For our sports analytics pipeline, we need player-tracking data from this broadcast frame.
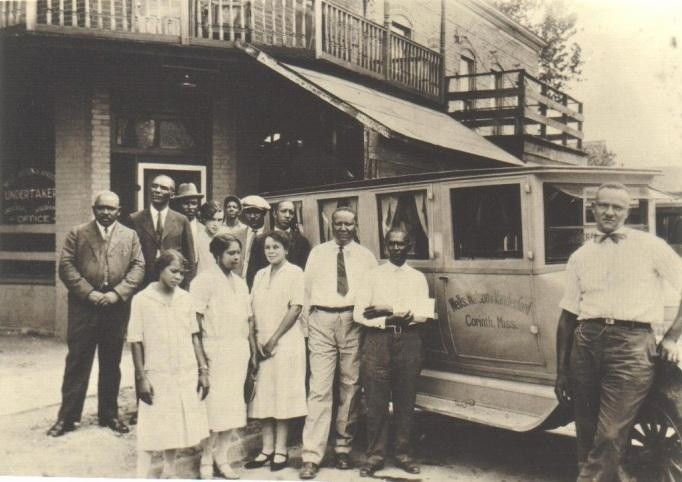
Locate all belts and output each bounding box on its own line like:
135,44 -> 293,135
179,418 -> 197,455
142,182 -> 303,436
581,315 -> 657,328
369,324 -> 423,335
309,303 -> 357,316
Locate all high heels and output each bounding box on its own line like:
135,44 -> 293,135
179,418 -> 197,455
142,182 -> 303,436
244,446 -> 275,471
268,450 -> 290,474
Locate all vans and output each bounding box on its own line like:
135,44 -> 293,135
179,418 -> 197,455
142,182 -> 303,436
244,166 -> 682,480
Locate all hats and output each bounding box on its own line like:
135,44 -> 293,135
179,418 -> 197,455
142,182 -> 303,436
238,194 -> 272,213
222,194 -> 242,215
171,182 -> 205,200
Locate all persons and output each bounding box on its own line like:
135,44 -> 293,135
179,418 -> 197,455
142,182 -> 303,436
555,182 -> 681,481
127,175 -> 378,478
353,226 -> 429,476
47,190 -> 143,437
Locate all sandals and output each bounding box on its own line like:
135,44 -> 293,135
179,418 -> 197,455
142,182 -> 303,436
199,458 -> 240,480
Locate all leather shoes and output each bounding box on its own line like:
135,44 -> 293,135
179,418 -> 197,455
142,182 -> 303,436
392,457 -> 421,475
332,452 -> 354,470
98,412 -> 130,435
298,461 -> 320,481
359,459 -> 385,478
46,420 -> 77,438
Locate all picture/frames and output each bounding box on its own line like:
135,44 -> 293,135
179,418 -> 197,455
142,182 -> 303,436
137,162 -> 207,212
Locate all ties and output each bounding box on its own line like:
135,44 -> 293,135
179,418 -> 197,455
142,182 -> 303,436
245,229 -> 258,264
154,211 -> 163,249
99,226 -> 111,288
336,245 -> 349,299
